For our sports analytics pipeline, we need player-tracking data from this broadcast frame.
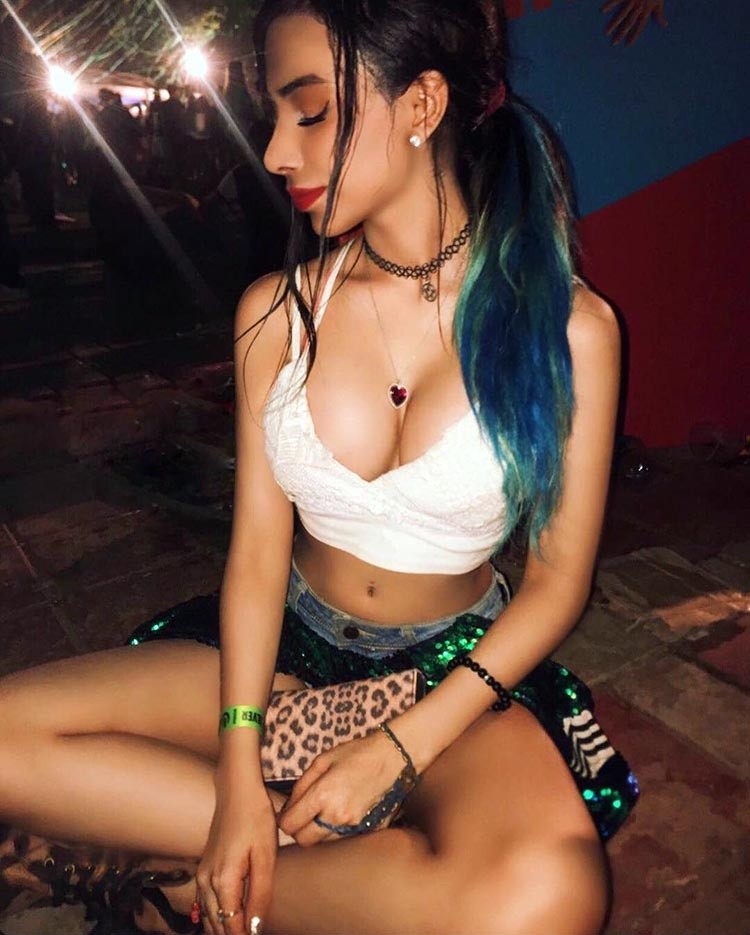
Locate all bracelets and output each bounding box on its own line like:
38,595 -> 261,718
447,655 -> 512,711
217,704 -> 265,735
375,720 -> 421,789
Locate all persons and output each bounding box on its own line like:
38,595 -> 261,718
17,80 -> 221,240
0,1 -> 623,934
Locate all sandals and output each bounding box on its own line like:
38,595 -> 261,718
0,825 -> 203,935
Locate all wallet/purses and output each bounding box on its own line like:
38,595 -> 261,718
259,666 -> 425,790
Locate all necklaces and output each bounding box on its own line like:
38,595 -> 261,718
361,216 -> 475,302
361,237 -> 468,410
